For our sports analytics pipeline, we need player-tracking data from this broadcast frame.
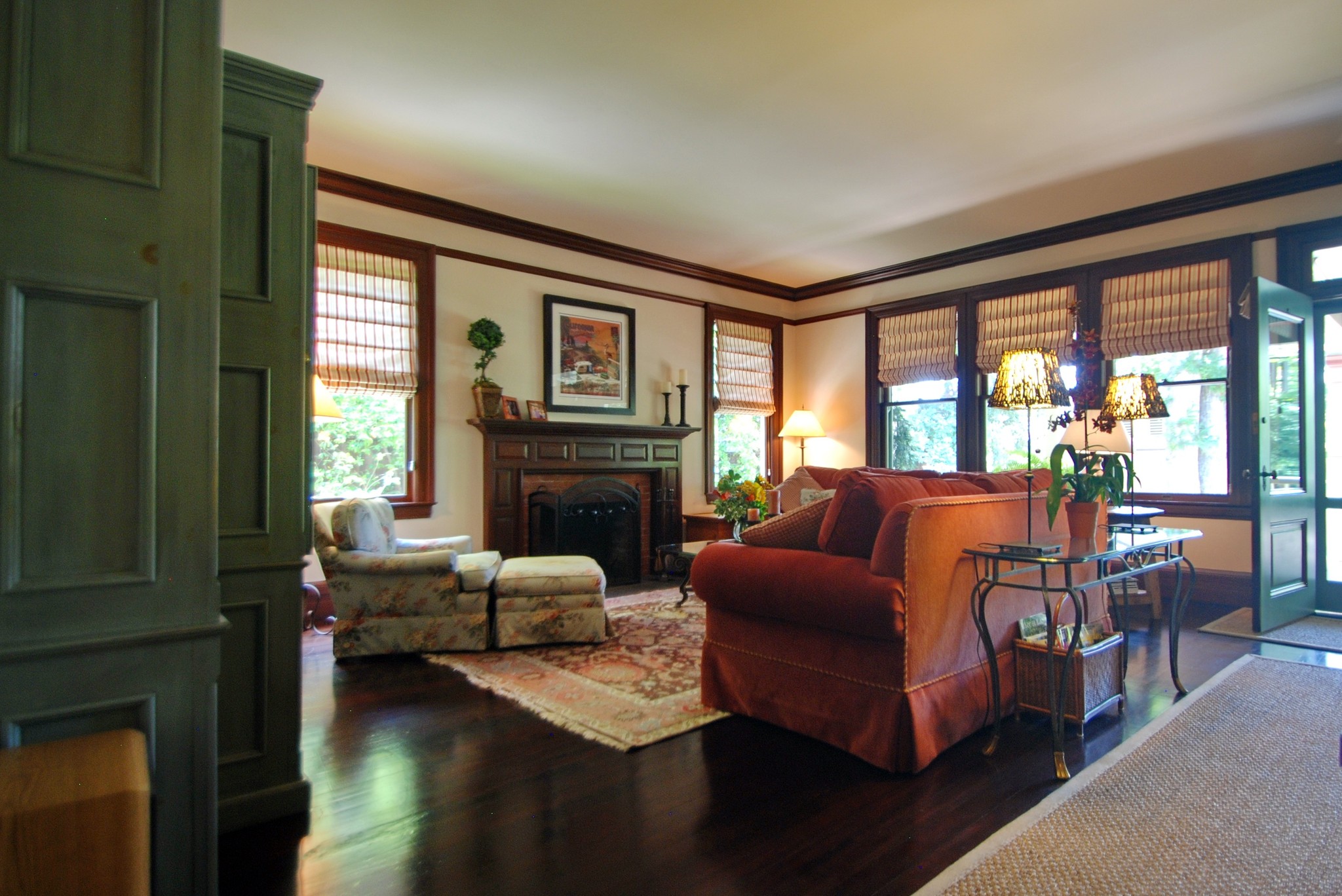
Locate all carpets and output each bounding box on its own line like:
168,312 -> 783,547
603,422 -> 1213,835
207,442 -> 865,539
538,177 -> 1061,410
1196,604 -> 1342,658
416,582 -> 736,754
910,650 -> 1341,896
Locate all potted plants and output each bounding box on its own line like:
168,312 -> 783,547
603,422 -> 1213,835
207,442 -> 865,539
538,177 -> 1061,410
468,314 -> 505,419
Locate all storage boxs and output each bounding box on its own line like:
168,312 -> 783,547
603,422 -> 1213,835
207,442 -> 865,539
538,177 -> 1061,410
1011,629 -> 1127,740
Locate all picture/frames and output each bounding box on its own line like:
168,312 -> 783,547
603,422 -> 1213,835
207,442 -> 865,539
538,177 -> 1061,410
501,396 -> 523,420
527,399 -> 549,422
542,293 -> 636,416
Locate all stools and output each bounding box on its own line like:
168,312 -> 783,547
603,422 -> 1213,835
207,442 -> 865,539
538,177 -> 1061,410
492,555 -> 615,650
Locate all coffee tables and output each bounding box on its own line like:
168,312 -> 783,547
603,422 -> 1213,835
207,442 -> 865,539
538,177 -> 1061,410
658,538 -> 736,608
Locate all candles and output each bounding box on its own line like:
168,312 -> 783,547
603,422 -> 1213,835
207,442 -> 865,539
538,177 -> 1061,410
679,369 -> 688,386
768,490 -> 780,515
747,508 -> 760,521
661,381 -> 672,393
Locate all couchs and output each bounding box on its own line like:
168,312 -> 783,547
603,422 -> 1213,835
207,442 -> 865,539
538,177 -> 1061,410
690,465 -> 1109,777
312,497 -> 501,658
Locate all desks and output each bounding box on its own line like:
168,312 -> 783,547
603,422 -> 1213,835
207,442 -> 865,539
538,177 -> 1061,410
961,525 -> 1205,780
1105,505 -> 1168,632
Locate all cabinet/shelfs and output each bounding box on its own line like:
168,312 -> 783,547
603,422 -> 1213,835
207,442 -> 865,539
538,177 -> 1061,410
683,512 -> 735,543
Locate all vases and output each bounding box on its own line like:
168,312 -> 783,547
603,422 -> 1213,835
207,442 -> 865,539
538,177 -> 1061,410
733,519 -> 751,543
1063,500 -> 1099,538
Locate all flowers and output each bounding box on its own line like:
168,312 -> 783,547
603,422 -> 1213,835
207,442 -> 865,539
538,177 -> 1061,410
1044,283 -> 1141,534
711,469 -> 776,523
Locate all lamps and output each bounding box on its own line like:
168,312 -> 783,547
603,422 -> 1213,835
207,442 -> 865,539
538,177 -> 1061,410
1097,372 -> 1169,533
987,345 -> 1064,554
777,410 -> 827,468
1058,410 -> 1134,476
310,373 -> 344,426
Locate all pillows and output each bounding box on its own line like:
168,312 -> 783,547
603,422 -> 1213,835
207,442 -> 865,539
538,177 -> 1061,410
772,465 -> 824,515
801,488 -> 836,506
739,497 -> 834,552
